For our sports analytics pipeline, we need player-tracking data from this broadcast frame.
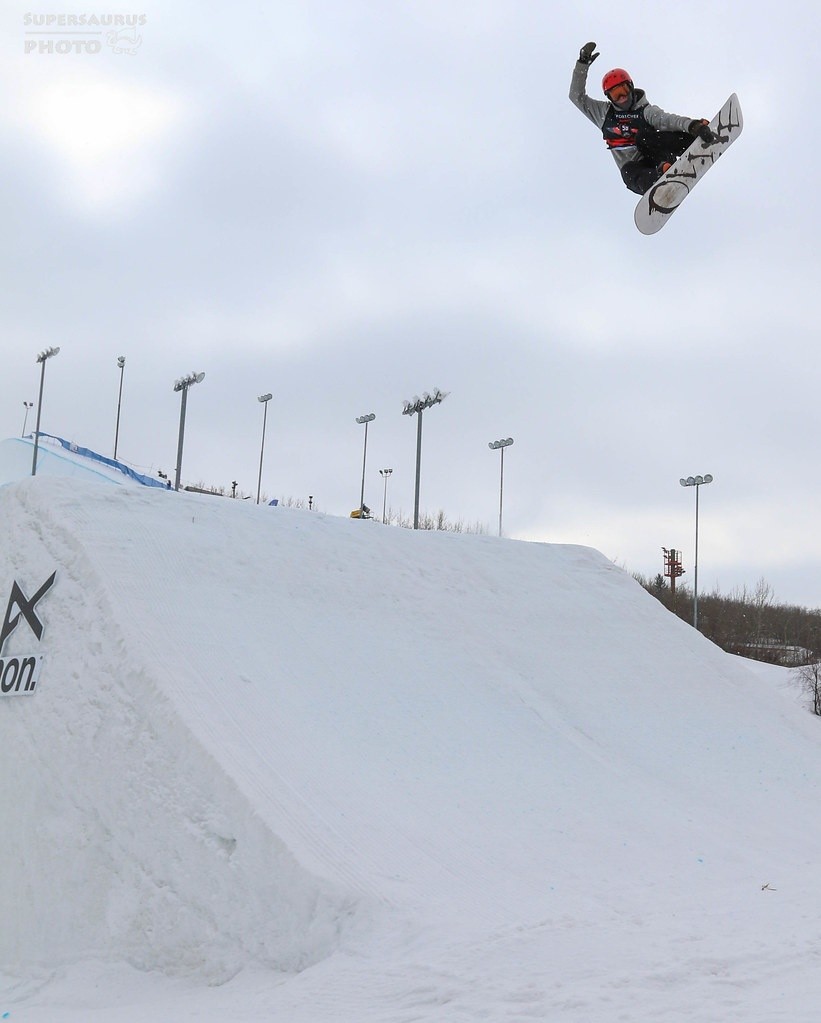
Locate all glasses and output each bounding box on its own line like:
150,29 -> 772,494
608,83 -> 631,102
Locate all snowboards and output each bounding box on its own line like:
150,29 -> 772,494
634,93 -> 744,235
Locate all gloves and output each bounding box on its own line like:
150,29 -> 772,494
577,42 -> 600,65
691,122 -> 714,143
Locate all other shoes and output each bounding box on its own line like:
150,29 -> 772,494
699,118 -> 711,126
656,161 -> 671,178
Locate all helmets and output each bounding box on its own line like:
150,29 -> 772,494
602,67 -> 634,96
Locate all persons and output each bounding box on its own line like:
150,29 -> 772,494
567,41 -> 719,198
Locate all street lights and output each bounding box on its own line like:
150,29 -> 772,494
257,393 -> 272,504
356,414 -> 375,509
22,400 -> 33,437
488,438 -> 514,538
32,346 -> 61,476
679,474 -> 713,626
173,372 -> 206,491
113,356 -> 125,459
402,386 -> 451,530
